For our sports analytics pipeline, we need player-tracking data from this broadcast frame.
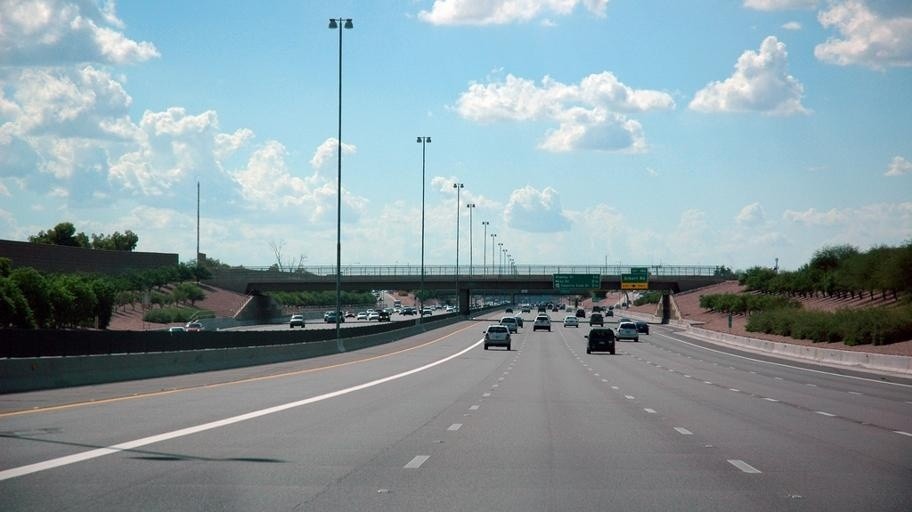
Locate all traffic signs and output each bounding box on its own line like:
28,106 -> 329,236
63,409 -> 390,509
621,274 -> 648,289
631,267 -> 647,274
553,274 -> 602,289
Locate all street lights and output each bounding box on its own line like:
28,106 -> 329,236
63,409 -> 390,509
453,183 -> 464,321
491,233 -> 496,311
481,220 -> 490,314
327,17 -> 355,352
502,247 -> 520,301
497,242 -> 503,309
467,203 -> 475,321
415,136 -> 431,333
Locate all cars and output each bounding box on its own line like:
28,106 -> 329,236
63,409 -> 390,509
289,300 -> 459,328
483,301 -> 649,356
168,327 -> 186,333
185,322 -> 205,332
